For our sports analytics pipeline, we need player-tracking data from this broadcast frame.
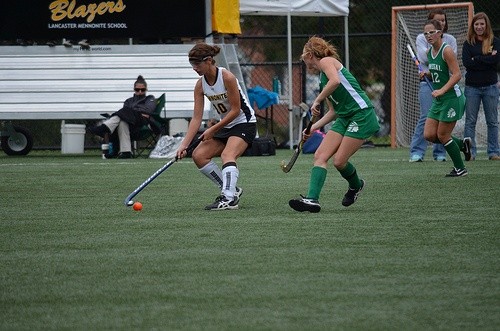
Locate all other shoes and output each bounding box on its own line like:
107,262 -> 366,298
118,152 -> 130,159
85,124 -> 107,138
410,155 -> 423,162
437,157 -> 447,161
489,154 -> 500,160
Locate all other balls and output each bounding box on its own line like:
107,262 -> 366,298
133,202 -> 142,210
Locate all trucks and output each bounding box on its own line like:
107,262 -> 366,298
0,0 -> 249,157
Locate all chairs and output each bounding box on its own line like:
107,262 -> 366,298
132,93 -> 167,158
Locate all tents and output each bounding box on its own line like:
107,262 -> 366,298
213,0 -> 349,148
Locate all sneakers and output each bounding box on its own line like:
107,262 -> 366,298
462,137 -> 472,161
445,167 -> 468,177
205,187 -> 243,211
289,194 -> 321,213
341,179 -> 366,207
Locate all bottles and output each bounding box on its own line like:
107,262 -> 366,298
278,80 -> 281,96
271,76 -> 278,94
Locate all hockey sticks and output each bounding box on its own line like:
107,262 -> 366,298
124,138 -> 202,207
407,43 -> 440,102
280,104 -> 321,174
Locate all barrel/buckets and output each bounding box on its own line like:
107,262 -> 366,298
60,124 -> 86,154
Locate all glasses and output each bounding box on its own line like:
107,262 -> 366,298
134,88 -> 146,92
301,52 -> 311,60
423,30 -> 442,36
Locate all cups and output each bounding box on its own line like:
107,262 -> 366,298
102,144 -> 109,159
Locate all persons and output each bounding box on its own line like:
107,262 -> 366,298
288,38 -> 379,213
304,8 -> 500,176
175,43 -> 258,212
87,75 -> 158,159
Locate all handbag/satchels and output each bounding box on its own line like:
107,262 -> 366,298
248,136 -> 276,157
